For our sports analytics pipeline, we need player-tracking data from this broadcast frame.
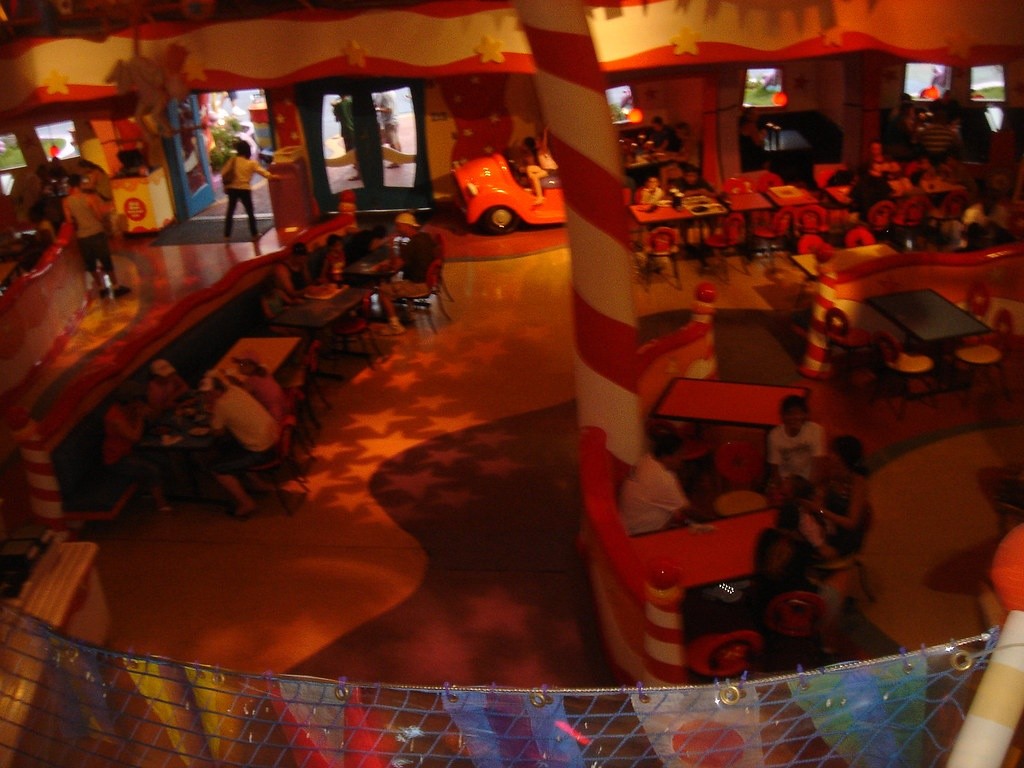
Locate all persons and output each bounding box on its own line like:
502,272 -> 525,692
851,142 -> 900,216
192,349 -> 292,518
765,394 -> 825,501
525,125 -> 552,205
222,141 -> 272,239
0,160 -> 111,261
62,174 -> 130,296
739,107 -> 770,170
749,435 -> 873,625
334,95 -> 363,181
373,91 -> 401,167
624,116 -> 713,250
958,190 -> 1006,252
262,215 -> 437,334
892,89 -> 967,167
617,433 -> 712,534
104,360 -> 187,510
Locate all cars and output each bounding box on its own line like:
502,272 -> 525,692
447,150 -> 633,236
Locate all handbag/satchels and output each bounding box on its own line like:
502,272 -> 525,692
221,159 -> 236,185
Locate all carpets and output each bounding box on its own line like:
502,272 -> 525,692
150,219 -> 273,246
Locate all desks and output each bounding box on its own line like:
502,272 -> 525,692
135,336 -> 303,515
823,179 -> 965,211
765,188 -> 819,251
651,377 -> 812,488
867,288 -> 992,399
1,543 -> 110,768
0,229 -> 37,261
268,286 -> 376,380
721,192 -> 776,260
341,245 -> 430,323
0,262 -> 21,286
628,196 -> 728,269
629,505 -> 808,588
760,128 -> 811,154
626,151 -> 675,168
40,192 -> 69,221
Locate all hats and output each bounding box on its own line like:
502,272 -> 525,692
291,242 -> 307,256
394,212 -> 422,228
150,359 -> 176,378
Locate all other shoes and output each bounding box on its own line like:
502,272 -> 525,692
113,284 -> 130,297
232,500 -> 263,520
99,287 -> 110,298
376,326 -> 406,335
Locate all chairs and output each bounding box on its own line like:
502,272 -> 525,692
687,590 -> 826,681
951,282 -> 1015,406
644,420 -> 768,519
810,500 -> 876,604
824,306 -> 935,421
623,171 -> 827,291
330,290 -> 385,371
237,339 -> 332,518
405,234 -> 455,334
814,162 -> 971,248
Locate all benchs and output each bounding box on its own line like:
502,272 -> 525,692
46,231 -> 373,520
